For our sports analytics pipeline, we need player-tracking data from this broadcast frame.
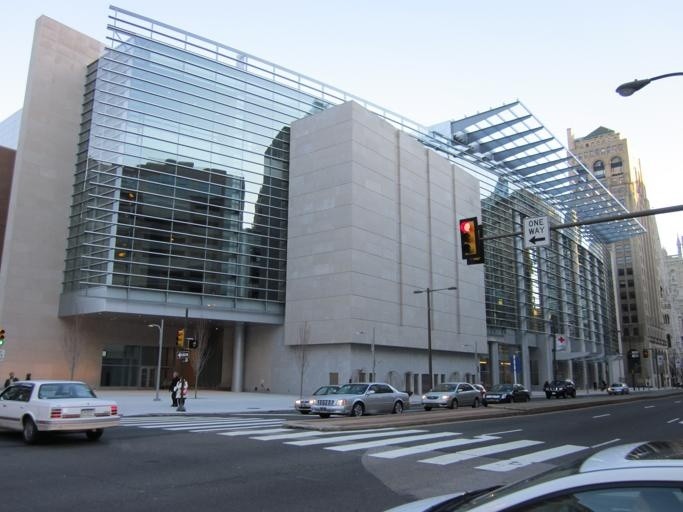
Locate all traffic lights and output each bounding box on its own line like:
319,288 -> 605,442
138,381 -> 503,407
0,329 -> 6,346
189,339 -> 198,348
458,215 -> 479,259
175,328 -> 184,350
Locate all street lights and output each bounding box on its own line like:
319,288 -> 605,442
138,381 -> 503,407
615,70 -> 681,99
413,285 -> 457,389
147,318 -> 164,400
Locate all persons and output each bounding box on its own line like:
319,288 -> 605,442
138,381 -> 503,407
168,371 -> 180,407
544,381 -> 550,400
5,371 -> 19,388
173,377 -> 188,408
26,373 -> 32,380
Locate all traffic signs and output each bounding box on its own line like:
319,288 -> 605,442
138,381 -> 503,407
522,215 -> 550,250
175,350 -> 189,360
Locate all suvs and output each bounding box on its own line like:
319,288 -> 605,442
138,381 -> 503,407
543,378 -> 576,399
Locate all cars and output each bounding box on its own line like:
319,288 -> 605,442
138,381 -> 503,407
379,437 -> 681,512
606,382 -> 628,394
0,379 -> 122,444
310,382 -> 409,418
472,382 -> 486,403
294,384 -> 344,414
482,383 -> 531,404
419,381 -> 483,410
